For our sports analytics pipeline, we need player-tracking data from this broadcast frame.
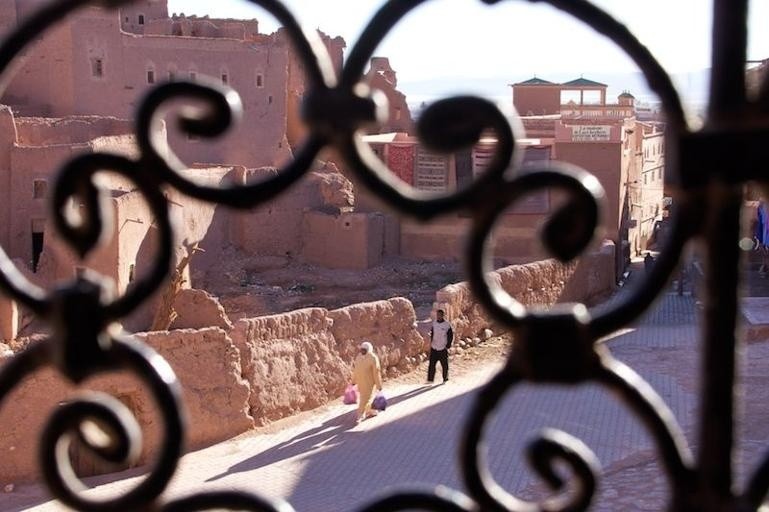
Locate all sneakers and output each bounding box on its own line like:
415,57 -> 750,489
441,379 -> 449,385
354,415 -> 364,426
367,410 -> 379,416
423,381 -> 435,385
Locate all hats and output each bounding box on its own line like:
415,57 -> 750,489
361,340 -> 373,349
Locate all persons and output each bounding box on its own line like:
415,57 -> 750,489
351,341 -> 384,425
643,252 -> 654,273
422,309 -> 453,384
